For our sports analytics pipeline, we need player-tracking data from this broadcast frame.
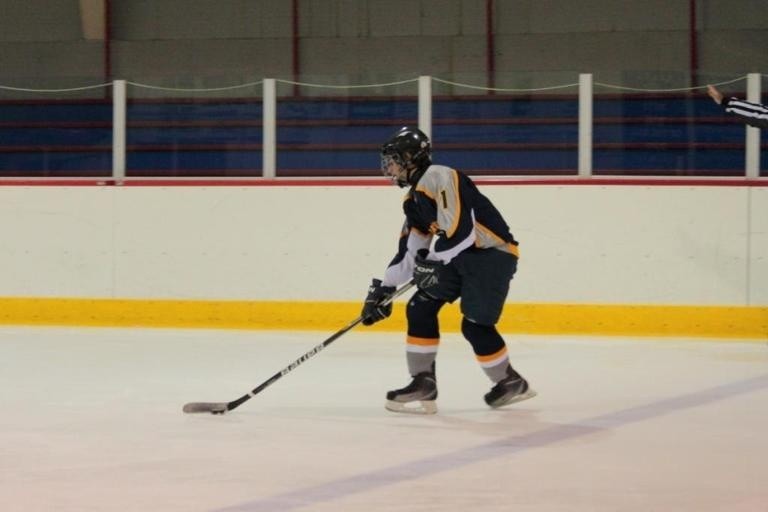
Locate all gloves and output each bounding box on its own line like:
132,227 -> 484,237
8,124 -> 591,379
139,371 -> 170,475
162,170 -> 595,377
361,278 -> 395,325
413,248 -> 443,290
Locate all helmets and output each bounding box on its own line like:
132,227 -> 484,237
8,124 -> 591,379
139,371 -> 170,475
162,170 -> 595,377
379,126 -> 429,182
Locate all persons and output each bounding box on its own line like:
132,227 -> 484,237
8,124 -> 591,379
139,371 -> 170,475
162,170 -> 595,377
706,84 -> 768,130
361,126 -> 528,408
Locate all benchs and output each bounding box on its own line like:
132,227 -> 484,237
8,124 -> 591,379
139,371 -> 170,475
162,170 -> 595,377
1,95 -> 765,175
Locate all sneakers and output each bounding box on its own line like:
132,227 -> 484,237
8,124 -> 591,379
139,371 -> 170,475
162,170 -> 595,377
484,365 -> 527,407
387,361 -> 436,403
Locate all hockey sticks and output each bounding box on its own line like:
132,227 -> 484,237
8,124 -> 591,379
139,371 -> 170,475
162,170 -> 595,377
183,280 -> 413,416
